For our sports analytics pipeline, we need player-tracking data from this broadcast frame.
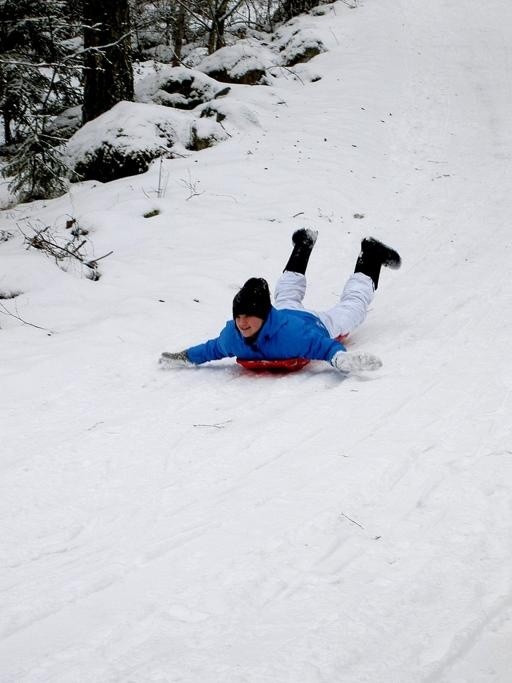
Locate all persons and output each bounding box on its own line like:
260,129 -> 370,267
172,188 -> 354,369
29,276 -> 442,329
151,221 -> 401,376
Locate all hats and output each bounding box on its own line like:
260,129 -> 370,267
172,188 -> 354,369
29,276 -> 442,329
231,276 -> 272,321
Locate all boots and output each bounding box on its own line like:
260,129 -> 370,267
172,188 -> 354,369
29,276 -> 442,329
282,227 -> 320,274
353,236 -> 402,289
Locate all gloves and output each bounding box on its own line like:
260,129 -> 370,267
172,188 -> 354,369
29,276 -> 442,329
161,349 -> 195,369
330,350 -> 384,374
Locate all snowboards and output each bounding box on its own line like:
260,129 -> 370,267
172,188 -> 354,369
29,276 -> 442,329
236,333 -> 350,371
